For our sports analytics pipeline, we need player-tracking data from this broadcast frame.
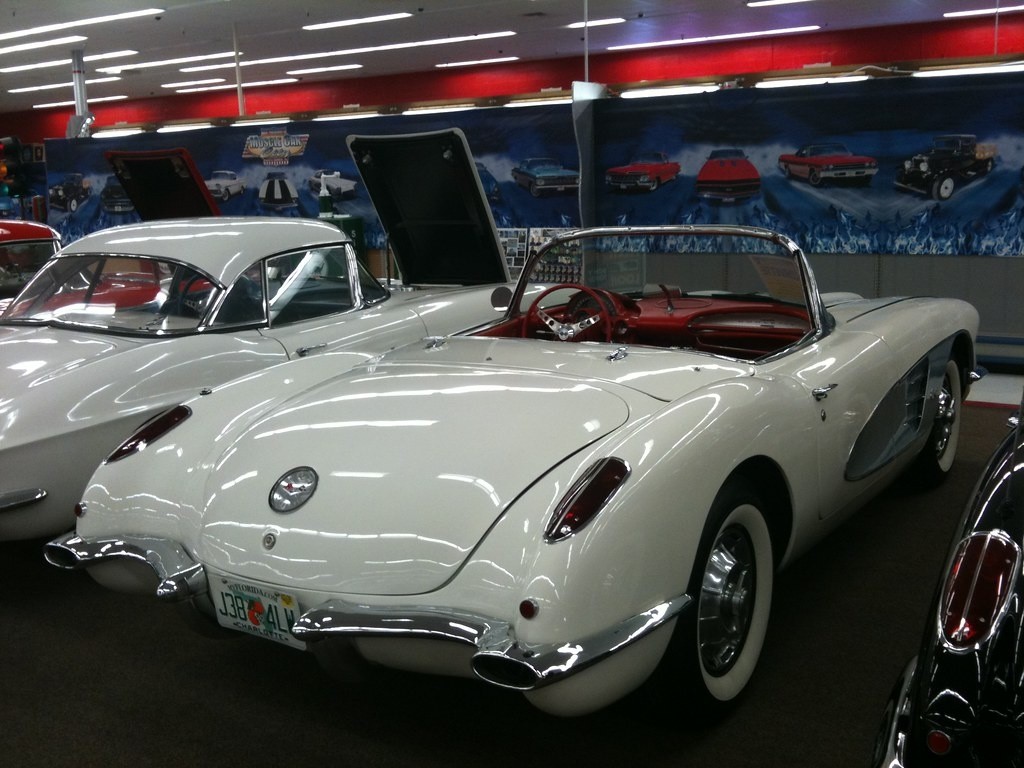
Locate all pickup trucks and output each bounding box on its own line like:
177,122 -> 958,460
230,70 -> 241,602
205,170 -> 247,202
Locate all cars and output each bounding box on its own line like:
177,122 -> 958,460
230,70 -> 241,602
510,157 -> 581,198
891,134 -> 996,203
605,151 -> 681,191
308,168 -> 359,201
0,146 -> 223,321
49,173 -> 94,212
258,172 -> 300,210
777,142 -> 882,188
474,161 -> 502,203
871,392 -> 1024,768
694,148 -> 762,203
100,175 -> 135,213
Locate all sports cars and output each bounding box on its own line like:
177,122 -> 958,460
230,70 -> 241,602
1,126 -> 583,545
42,217 -> 988,727
